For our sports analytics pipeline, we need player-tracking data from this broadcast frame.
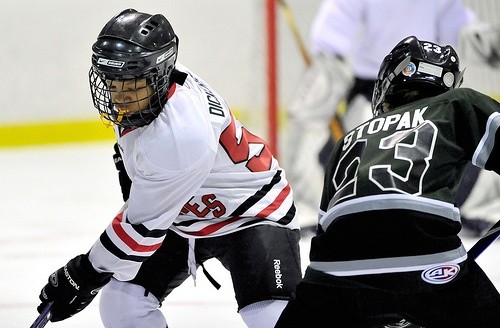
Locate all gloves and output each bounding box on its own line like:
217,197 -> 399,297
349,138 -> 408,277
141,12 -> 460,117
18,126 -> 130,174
37,252 -> 114,323
114,142 -> 131,202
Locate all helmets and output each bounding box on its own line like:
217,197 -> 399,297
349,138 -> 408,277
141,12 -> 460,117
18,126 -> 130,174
371,36 -> 465,118
91,8 -> 178,128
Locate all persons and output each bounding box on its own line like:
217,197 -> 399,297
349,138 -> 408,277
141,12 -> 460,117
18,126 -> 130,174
38,8 -> 302,328
273,37 -> 500,328
306,0 -> 500,173
